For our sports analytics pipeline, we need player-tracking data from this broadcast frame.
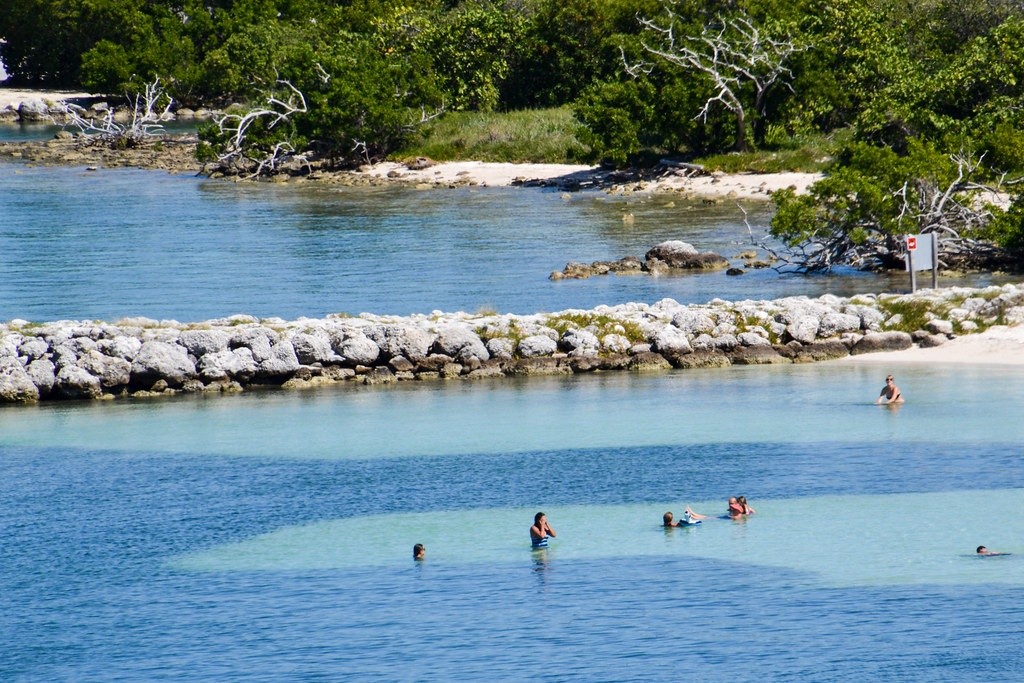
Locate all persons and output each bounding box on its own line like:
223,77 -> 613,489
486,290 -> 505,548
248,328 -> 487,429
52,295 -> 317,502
530,511 -> 556,548
875,375 -> 904,403
413,544 -> 425,559
976,545 -> 991,553
663,496 -> 755,526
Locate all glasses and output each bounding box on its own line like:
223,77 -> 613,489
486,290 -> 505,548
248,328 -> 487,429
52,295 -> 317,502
886,378 -> 893,381
420,548 -> 426,552
